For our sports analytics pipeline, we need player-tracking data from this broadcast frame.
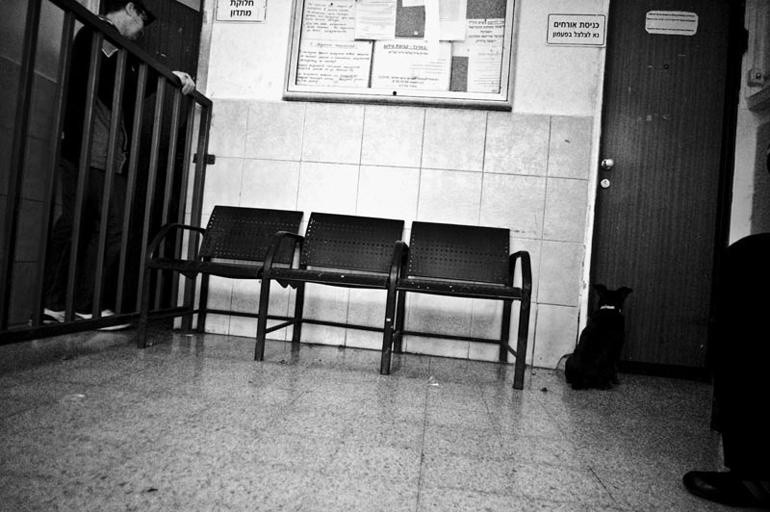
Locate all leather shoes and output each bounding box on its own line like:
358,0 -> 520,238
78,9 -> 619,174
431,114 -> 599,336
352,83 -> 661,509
682,467 -> 770,511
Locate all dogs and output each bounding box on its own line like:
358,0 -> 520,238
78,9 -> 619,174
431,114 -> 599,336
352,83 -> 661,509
564,284 -> 634,391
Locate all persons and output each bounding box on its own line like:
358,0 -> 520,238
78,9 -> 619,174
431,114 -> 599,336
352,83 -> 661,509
25,0 -> 197,331
681,228 -> 769,511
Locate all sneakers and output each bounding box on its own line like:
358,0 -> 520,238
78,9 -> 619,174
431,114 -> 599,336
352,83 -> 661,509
27,307 -> 65,327
74,311 -> 130,332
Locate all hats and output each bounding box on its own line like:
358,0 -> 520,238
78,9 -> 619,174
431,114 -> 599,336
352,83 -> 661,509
102,0 -> 156,26
